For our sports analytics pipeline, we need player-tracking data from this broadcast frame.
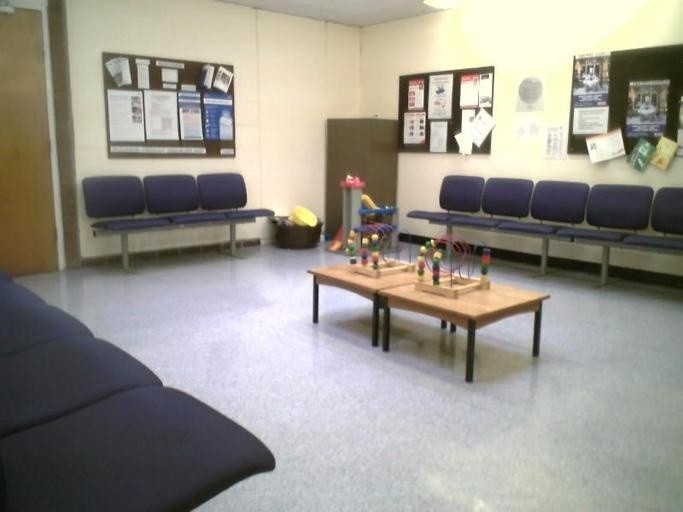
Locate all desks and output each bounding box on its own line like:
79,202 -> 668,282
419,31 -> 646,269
376,271 -> 550,382
306,257 -> 456,347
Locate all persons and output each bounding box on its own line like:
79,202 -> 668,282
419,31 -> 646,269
581,66 -> 605,94
635,95 -> 660,123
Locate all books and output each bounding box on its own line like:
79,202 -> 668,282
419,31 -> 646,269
651,136 -> 678,170
626,137 -> 657,171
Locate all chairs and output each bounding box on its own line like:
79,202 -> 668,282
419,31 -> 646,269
405,174 -> 682,289
82,172 -> 275,275
0,268 -> 275,511
